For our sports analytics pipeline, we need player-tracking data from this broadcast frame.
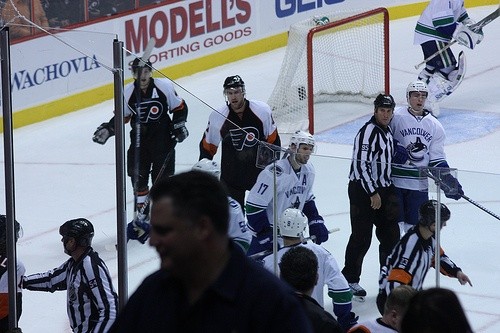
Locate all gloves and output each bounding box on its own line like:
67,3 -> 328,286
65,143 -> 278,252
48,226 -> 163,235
338,312 -> 359,329
441,175 -> 464,200
126,220 -> 149,245
93,122 -> 116,144
308,216 -> 329,245
259,231 -> 279,251
172,121 -> 189,142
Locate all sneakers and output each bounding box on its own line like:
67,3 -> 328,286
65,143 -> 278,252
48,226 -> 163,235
348,282 -> 366,302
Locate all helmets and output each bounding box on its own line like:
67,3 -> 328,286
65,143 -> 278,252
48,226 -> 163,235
406,82 -> 429,94
291,131 -> 315,146
374,93 -> 396,106
0,216 -> 20,244
131,58 -> 152,71
192,158 -> 221,178
420,200 -> 451,220
59,218 -> 94,240
280,208 -> 308,237
224,76 -> 245,90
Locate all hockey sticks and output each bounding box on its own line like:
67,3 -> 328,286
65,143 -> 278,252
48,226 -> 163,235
409,161 -> 500,220
414,6 -> 500,72
249,227 -> 340,260
134,135 -> 180,232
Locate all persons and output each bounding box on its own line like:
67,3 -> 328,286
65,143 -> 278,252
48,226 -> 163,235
0,215 -> 24,333
108,170 -> 311,332
377,199 -> 473,317
196,73 -> 280,202
189,159 -> 252,256
380,81 -> 462,234
342,94 -> 400,304
271,248 -> 338,333
21,217 -> 118,333
91,56 -> 189,225
415,1 -> 484,101
401,288 -> 473,333
346,285 -> 416,333
260,209 -> 353,320
244,128 -> 330,259
